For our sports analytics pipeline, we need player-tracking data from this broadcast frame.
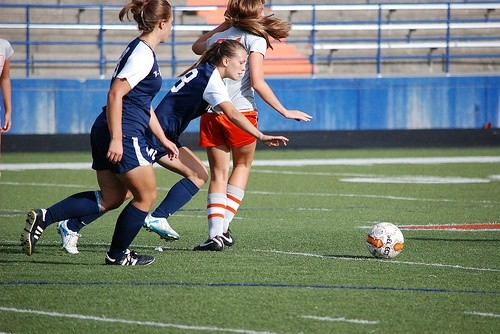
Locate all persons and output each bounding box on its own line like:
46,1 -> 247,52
57,39 -> 290,254
0,36 -> 15,135
192,0 -> 313,251
19,0 -> 183,268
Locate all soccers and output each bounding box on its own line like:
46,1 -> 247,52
367,222 -> 404,259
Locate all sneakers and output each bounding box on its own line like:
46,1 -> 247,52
106,249 -> 156,266
20,208 -> 47,256
191,235 -> 225,251
143,213 -> 180,243
56,219 -> 83,255
221,228 -> 235,246
